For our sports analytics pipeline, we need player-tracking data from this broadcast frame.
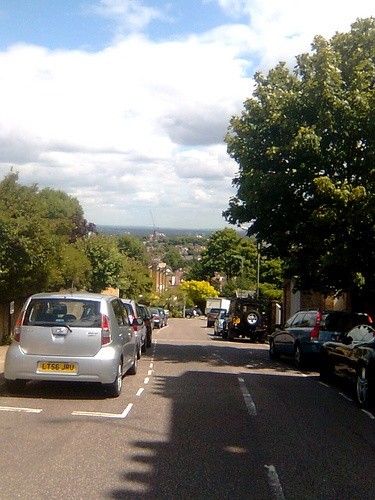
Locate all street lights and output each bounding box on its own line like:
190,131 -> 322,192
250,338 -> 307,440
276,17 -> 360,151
241,226 -> 261,298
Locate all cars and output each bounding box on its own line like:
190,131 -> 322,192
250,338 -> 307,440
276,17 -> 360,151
4,293 -> 137,396
205,297 -> 231,335
185,308 -> 201,318
119,298 -> 169,359
319,323 -> 375,408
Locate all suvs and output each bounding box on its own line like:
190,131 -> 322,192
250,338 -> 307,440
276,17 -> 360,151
270,308 -> 375,371
222,298 -> 272,343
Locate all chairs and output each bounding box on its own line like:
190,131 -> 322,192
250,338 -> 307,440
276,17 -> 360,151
35,312 -> 56,322
62,315 -> 76,322
